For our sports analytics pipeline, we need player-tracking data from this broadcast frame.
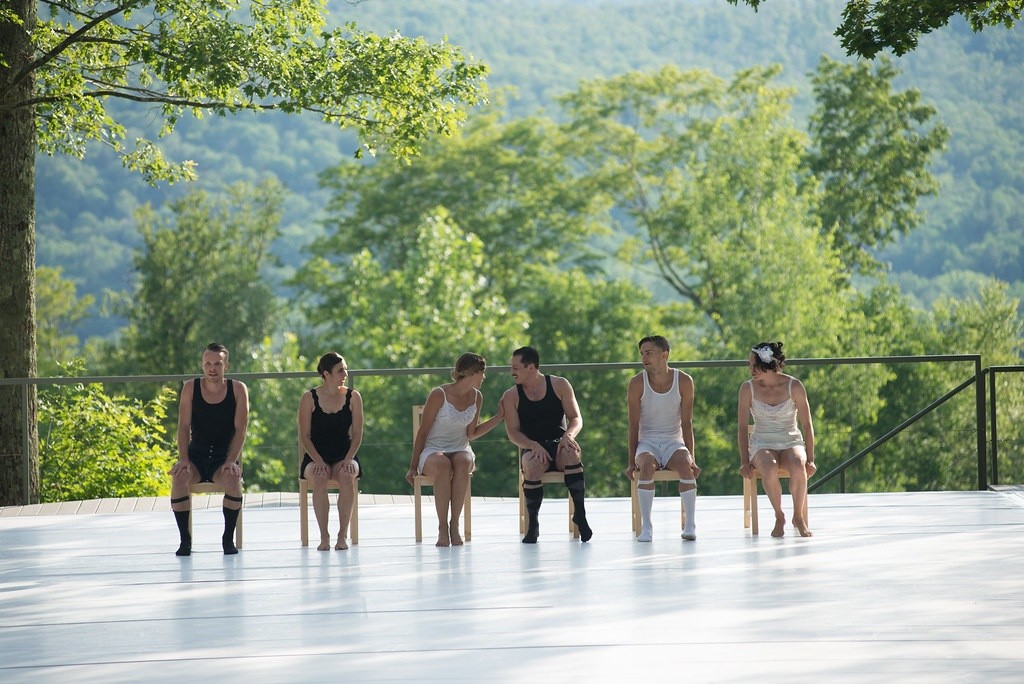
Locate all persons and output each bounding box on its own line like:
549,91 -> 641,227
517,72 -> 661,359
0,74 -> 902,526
405,352 -> 506,548
298,352 -> 364,551
166,342 -> 250,556
737,340 -> 817,538
502,345 -> 593,543
623,335 -> 701,542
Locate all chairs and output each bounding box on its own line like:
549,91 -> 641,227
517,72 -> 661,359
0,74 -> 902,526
518,446 -> 580,539
627,468 -> 696,538
742,423 -> 810,535
297,409 -> 361,546
183,445 -> 245,549
411,405 -> 474,543
